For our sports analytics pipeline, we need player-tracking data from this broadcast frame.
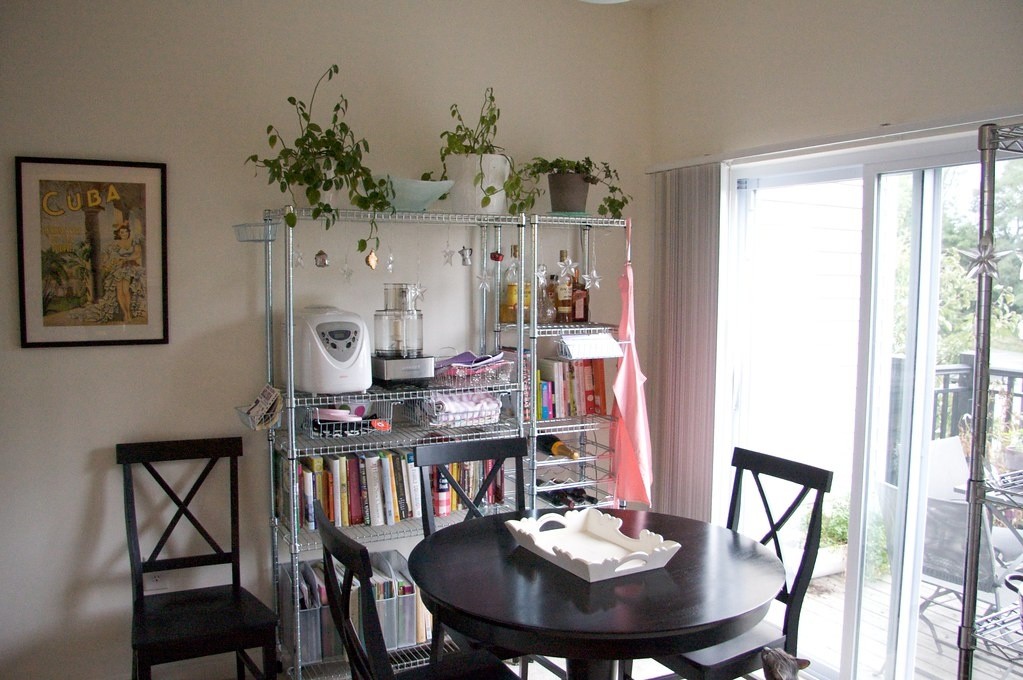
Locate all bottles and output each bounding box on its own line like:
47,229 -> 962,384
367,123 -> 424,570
500,244 -> 589,324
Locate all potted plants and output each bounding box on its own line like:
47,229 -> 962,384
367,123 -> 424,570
421,87 -> 546,215
244,65 -> 397,252
522,156 -> 633,221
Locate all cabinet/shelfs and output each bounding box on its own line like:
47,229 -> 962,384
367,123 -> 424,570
231,205 -> 629,680
957,122 -> 1022,679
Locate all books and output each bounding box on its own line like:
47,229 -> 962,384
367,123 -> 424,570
274,440 -> 513,536
280,560 -> 433,664
499,345 -> 612,422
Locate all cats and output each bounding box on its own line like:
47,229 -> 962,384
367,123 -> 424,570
761,645 -> 810,680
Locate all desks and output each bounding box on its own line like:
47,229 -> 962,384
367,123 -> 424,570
408,508 -> 787,680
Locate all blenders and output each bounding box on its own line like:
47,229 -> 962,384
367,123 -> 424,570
373,282 -> 435,385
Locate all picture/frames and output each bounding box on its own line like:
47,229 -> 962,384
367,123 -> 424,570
13,155 -> 168,348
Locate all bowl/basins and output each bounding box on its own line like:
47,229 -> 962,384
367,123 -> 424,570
361,172 -> 456,212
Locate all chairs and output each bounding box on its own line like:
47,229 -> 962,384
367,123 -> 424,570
878,479 -> 1023,619
413,437 -> 569,680
116,438 -> 279,679
319,522 -> 523,680
617,447 -> 832,680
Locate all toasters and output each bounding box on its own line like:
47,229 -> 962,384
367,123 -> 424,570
279,304 -> 373,396
